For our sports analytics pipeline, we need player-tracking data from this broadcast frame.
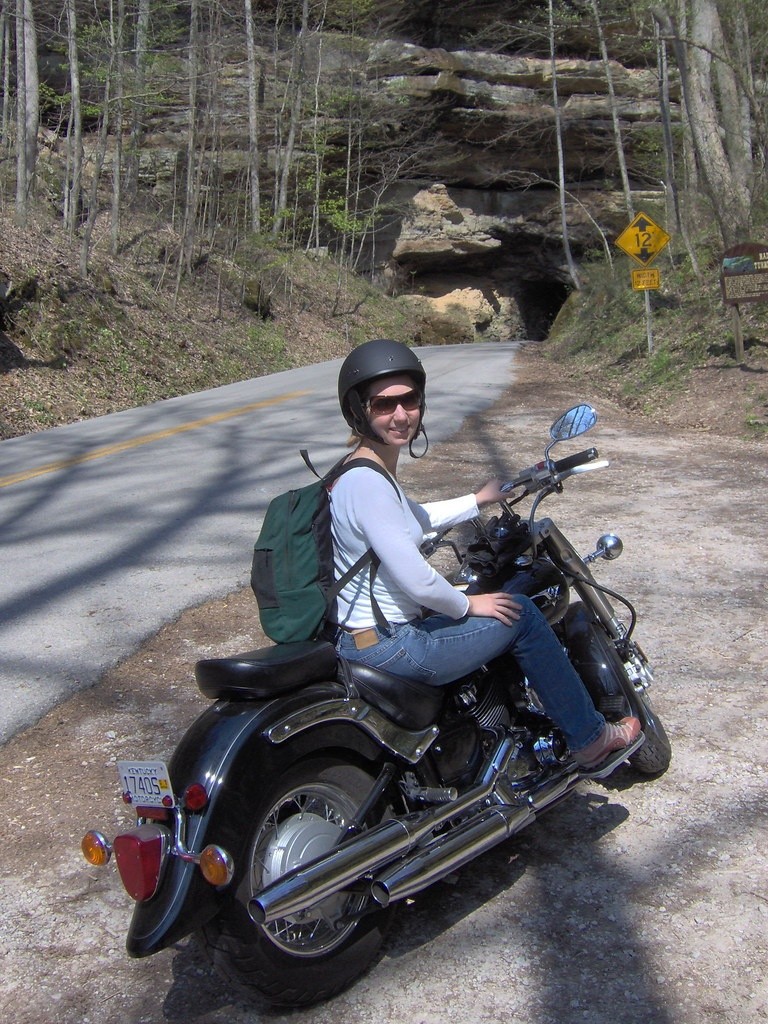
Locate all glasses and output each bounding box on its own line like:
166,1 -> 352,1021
364,385 -> 422,415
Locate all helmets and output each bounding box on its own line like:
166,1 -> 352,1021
338,339 -> 426,429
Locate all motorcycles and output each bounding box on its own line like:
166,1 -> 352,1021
81,403 -> 672,1006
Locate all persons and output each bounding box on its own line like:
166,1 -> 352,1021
322,340 -> 640,769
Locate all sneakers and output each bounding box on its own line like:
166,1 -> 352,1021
572,717 -> 641,770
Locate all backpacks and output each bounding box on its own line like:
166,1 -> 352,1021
250,450 -> 402,645
